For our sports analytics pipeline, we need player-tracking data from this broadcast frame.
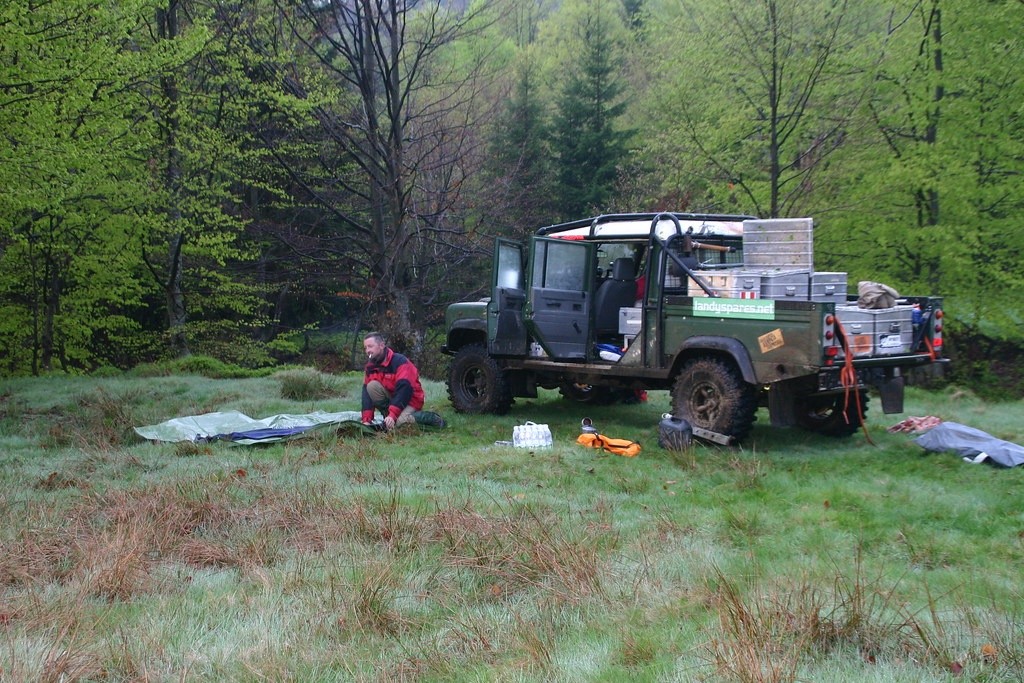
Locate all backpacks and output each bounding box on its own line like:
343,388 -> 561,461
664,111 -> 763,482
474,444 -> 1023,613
575,434 -> 642,458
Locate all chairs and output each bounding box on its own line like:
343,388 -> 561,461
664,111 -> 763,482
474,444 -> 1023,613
594,257 -> 638,337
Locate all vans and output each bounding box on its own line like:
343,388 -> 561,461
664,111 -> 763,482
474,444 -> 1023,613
535,210 -> 762,297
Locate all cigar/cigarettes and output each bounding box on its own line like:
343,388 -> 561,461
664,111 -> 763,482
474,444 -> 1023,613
369,353 -> 372,359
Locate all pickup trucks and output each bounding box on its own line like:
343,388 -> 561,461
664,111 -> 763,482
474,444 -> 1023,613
437,210 -> 954,447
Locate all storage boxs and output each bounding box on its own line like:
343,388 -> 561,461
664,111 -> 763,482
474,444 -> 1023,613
688,217 -> 913,359
619,307 -> 642,335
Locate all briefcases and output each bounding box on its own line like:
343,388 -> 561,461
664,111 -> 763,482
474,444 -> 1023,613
512,421 -> 553,452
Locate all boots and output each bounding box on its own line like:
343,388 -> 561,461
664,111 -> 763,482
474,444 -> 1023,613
375,399 -> 390,419
411,411 -> 446,431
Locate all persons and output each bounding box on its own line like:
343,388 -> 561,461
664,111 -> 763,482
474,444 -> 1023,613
357,332 -> 445,432
